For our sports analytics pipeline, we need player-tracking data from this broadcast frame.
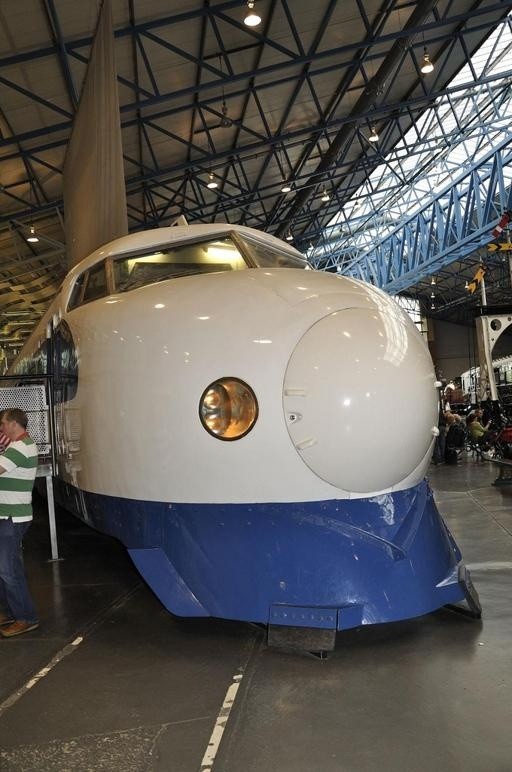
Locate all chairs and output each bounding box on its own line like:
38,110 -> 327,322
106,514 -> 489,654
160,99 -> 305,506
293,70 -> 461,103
436,395 -> 512,463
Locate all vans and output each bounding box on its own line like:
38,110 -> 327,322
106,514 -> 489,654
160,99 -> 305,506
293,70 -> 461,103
446,401 -> 478,417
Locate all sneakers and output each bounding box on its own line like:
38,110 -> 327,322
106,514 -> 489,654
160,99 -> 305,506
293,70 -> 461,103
0,612 -> 16,625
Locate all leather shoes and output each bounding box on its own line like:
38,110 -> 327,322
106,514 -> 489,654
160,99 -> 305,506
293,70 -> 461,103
0,617 -> 41,638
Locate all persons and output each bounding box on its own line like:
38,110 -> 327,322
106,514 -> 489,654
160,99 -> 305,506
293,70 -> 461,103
0,408 -> 43,636
433,403 -> 496,464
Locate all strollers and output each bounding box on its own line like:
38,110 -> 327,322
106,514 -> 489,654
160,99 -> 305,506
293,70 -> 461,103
482,402 -> 512,459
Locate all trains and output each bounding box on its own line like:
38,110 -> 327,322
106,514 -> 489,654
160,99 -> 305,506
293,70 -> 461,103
0,214 -> 480,660
